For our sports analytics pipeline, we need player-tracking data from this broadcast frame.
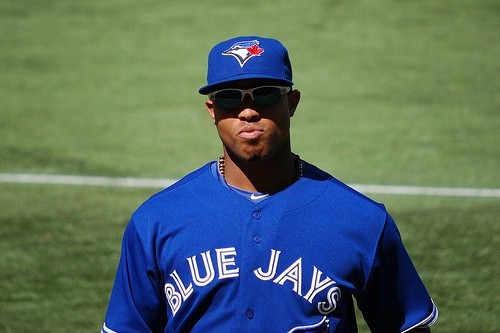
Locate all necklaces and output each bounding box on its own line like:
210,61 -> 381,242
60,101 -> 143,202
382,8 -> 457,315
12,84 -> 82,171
217,152 -> 307,191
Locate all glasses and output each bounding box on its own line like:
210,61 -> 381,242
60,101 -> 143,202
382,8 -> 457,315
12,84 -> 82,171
206,84 -> 289,107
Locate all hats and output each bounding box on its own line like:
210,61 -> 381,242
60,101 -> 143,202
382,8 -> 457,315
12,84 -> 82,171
197,36 -> 297,94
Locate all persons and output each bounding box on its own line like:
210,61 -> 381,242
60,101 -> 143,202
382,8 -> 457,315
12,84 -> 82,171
100,36 -> 441,333
